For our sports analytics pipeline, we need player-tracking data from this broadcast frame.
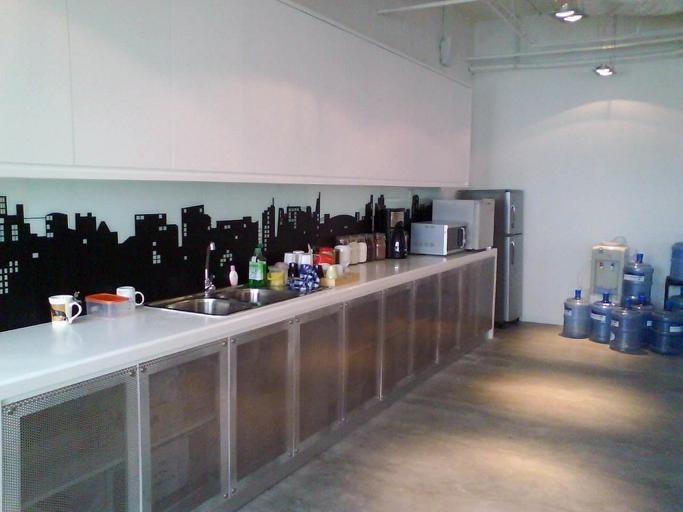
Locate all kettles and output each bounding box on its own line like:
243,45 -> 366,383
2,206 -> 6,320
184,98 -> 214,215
388,220 -> 408,259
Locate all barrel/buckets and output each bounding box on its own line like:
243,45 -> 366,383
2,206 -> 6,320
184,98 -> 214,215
590,292 -> 619,343
622,253 -> 655,306
563,289 -> 592,338
669,242 -> 683,283
669,287 -> 683,310
629,295 -> 656,347
649,299 -> 683,355
609,296 -> 643,353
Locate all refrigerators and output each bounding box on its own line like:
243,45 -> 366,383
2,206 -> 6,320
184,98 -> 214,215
456,186 -> 522,328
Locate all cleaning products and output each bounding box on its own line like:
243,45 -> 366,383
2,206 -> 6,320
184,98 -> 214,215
247,245 -> 268,288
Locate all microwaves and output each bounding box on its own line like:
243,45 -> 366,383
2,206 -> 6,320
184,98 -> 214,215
409,219 -> 468,258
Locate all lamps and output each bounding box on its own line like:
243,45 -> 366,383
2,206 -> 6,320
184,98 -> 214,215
592,62 -> 614,77
551,1 -> 585,23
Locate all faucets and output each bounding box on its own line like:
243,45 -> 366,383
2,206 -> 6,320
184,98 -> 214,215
203,241 -> 218,296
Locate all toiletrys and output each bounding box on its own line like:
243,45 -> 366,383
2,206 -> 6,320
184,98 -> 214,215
229,265 -> 238,286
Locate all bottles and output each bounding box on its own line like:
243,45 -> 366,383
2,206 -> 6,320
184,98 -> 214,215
228,265 -> 238,287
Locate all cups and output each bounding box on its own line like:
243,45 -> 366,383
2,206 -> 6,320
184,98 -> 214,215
48,294 -> 82,329
115,285 -> 144,313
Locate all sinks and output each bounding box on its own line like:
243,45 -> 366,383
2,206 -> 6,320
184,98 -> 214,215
217,285 -> 301,307
145,296 -> 255,317
